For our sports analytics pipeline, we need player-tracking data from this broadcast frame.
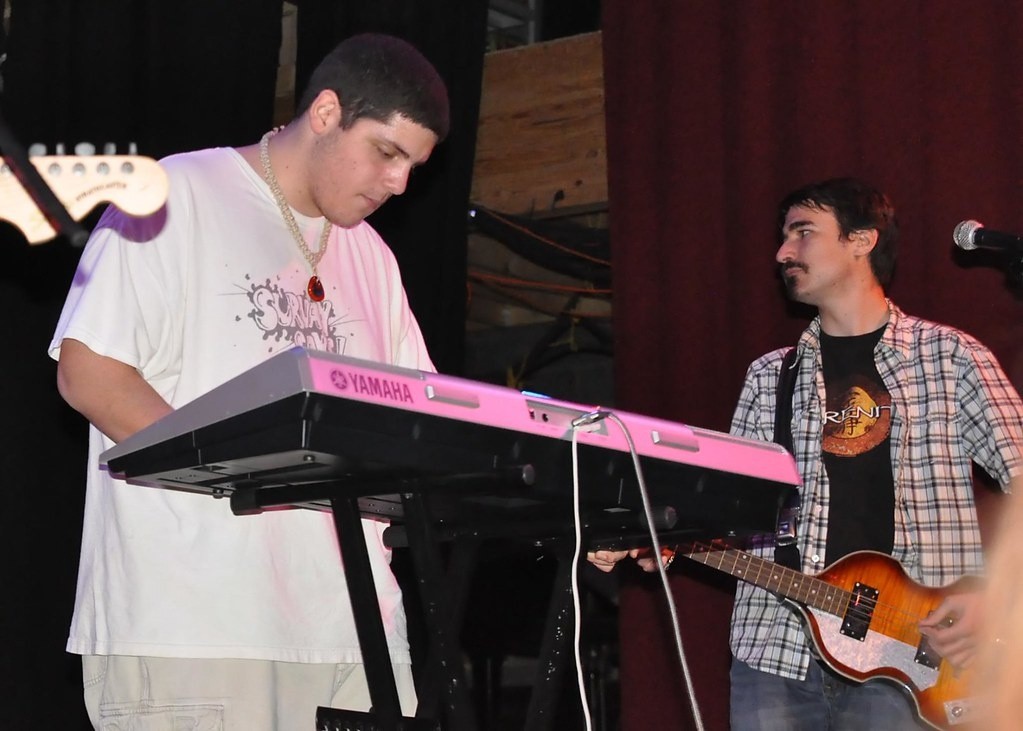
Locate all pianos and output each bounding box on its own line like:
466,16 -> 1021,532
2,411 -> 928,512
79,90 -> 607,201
98,345 -> 800,559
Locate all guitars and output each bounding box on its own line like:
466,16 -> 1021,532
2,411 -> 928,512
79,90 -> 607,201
640,534 -> 1023,731
0,144 -> 166,246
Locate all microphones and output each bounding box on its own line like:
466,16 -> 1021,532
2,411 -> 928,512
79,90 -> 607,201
952,220 -> 1023,255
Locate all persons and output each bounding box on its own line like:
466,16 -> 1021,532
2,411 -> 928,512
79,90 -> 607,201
585,175 -> 1023,731
47,32 -> 451,731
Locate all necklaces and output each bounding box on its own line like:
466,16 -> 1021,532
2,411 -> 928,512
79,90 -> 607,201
260,124 -> 334,303
